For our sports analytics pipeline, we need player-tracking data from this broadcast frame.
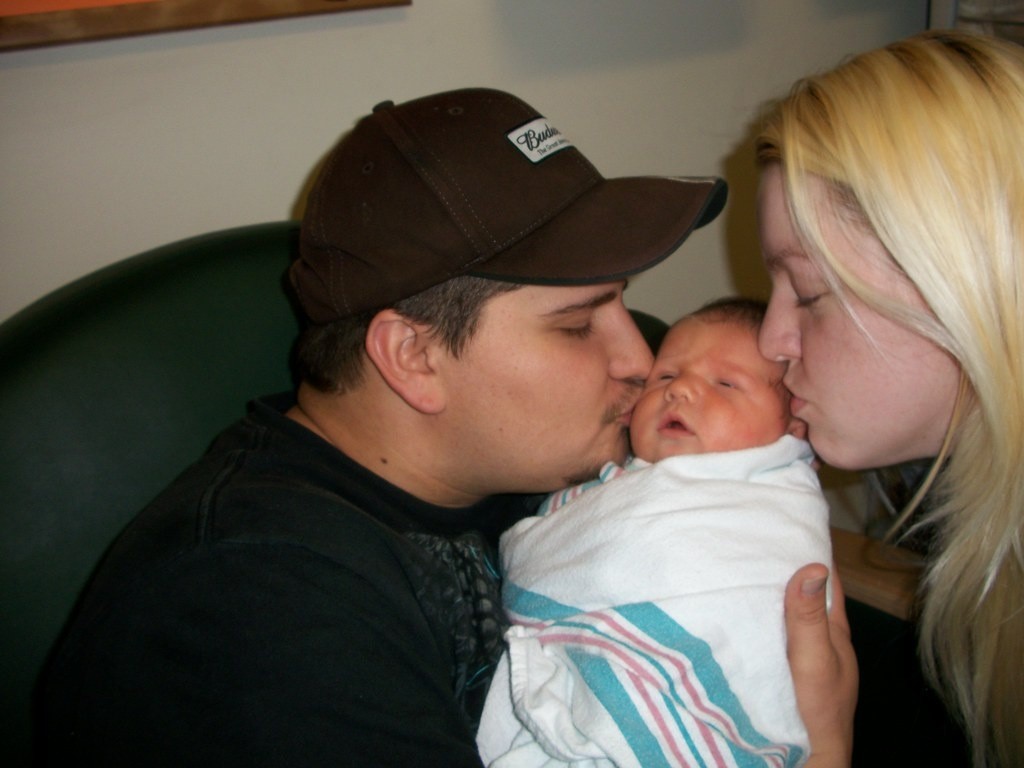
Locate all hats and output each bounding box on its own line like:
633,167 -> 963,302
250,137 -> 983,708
289,88 -> 728,326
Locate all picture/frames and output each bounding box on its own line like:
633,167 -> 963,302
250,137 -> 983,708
0,0 -> 413,54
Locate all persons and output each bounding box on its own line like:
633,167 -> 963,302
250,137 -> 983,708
741,30 -> 1024,768
32,89 -> 859,767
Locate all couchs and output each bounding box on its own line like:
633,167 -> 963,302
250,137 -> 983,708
0,219 -> 671,768
